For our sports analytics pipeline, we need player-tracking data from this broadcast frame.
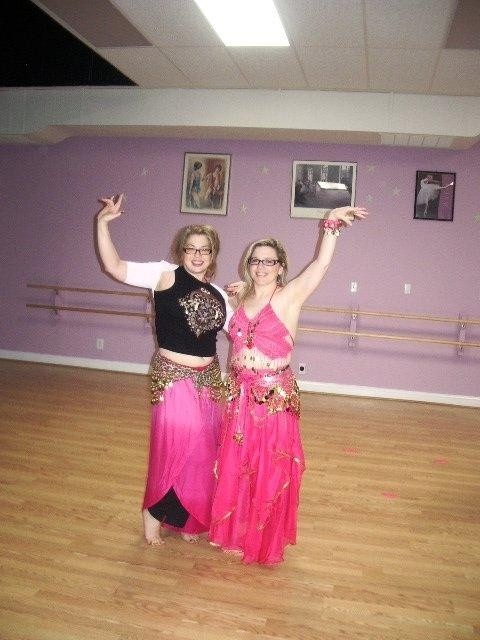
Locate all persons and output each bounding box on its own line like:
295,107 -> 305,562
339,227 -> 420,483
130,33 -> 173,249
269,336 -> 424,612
206,205 -> 368,569
95,193 -> 234,549
206,164 -> 223,210
188,161 -> 204,210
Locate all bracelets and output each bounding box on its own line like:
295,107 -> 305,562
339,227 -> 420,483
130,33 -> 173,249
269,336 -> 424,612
322,219 -> 342,237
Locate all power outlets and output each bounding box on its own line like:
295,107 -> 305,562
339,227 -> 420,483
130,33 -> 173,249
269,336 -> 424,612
351,282 -> 357,293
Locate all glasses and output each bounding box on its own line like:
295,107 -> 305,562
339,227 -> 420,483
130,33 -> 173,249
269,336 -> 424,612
183,247 -> 212,255
248,258 -> 281,266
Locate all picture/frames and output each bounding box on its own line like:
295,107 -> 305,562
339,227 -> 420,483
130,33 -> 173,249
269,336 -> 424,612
414,170 -> 456,222
180,152 -> 232,217
290,160 -> 357,221
